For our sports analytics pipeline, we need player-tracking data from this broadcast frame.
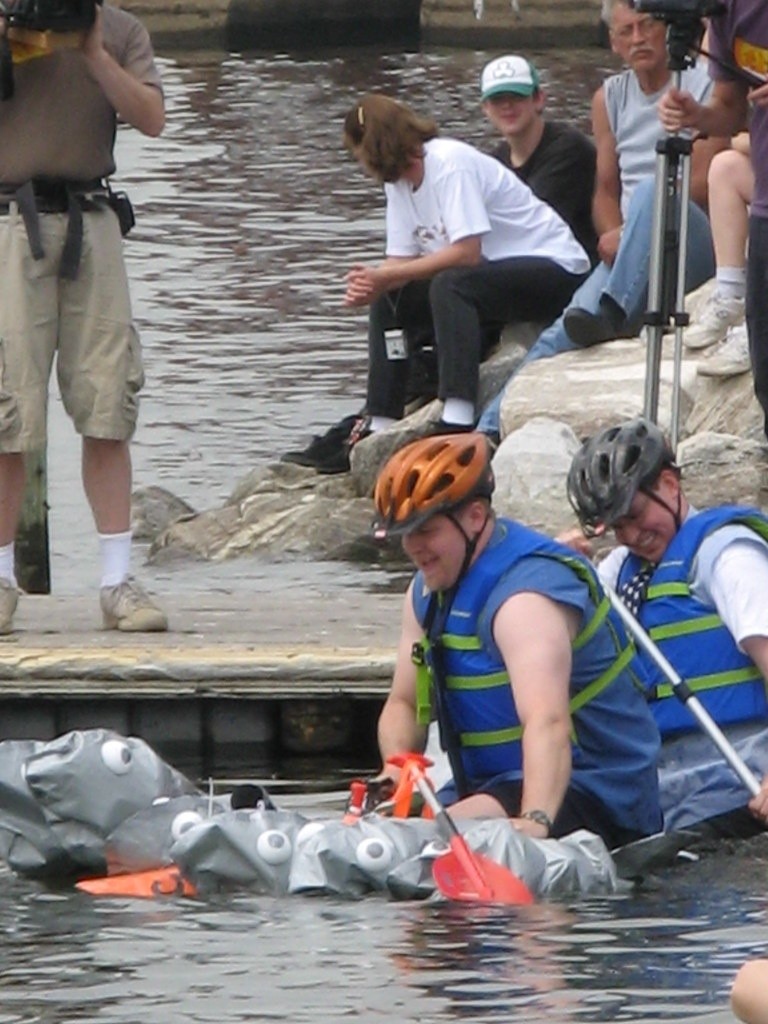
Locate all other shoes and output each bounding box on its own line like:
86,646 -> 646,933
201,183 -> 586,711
316,429 -> 375,473
280,432 -> 345,465
682,291 -> 749,350
697,323 -> 753,376
428,419 -> 475,435
564,308 -> 617,348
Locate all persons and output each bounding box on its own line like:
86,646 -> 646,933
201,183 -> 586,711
342,431 -> 663,849
564,420 -> 768,830
278,52 -> 597,472
0,1 -> 169,634
472,0 -> 715,442
316,91 -> 591,473
660,1 -> 767,440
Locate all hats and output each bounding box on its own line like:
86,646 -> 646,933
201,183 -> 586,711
479,53 -> 540,102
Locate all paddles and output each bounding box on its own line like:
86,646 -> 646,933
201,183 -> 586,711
600,583 -> 764,800
407,760 -> 534,905
74,759 -> 411,897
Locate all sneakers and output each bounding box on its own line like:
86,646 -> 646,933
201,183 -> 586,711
0,578 -> 19,635
100,574 -> 167,632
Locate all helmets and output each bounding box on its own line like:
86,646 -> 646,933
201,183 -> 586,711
566,418 -> 674,538
371,433 -> 488,539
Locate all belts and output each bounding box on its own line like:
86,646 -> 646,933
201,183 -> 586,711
0,199 -> 88,214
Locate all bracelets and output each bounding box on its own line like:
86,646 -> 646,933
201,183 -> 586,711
522,804 -> 554,836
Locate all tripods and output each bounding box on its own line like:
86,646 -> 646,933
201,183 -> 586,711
640,11 -> 768,463
